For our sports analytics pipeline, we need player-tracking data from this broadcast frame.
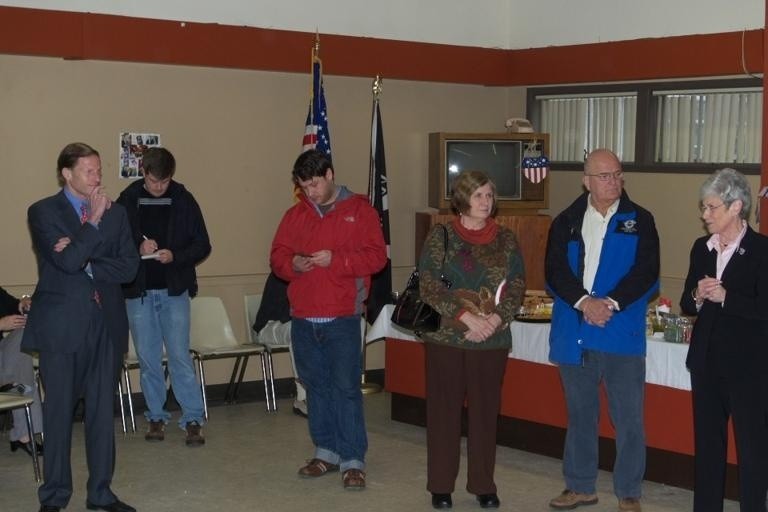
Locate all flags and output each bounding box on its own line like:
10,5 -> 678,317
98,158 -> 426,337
290,28 -> 333,198
357,73 -> 391,340
522,141 -> 548,182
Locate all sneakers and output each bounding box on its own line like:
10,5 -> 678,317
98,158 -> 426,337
549,489 -> 598,509
185,421 -> 205,446
145,421 -> 165,442
619,497 -> 641,512
298,459 -> 340,479
342,468 -> 365,491
293,400 -> 308,419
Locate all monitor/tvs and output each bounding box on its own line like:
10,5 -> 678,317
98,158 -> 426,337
428,132 -> 550,216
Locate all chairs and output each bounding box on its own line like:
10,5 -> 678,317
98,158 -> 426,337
233,294 -> 291,414
189,295 -> 270,421
124,328 -> 193,434
35,372 -> 127,435
0,393 -> 41,482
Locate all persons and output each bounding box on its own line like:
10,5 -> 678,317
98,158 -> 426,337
421,169 -> 524,508
253,271 -> 311,419
679,167 -> 768,512
21,143 -> 139,510
1,289 -> 43,458
117,147 -> 212,448
269,151 -> 386,492
548,149 -> 662,512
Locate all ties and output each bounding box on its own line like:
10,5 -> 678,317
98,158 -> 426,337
80,203 -> 102,310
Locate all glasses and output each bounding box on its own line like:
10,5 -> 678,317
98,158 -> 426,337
701,202 -> 728,212
585,171 -> 625,181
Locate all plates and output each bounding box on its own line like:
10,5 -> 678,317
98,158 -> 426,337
514,311 -> 552,323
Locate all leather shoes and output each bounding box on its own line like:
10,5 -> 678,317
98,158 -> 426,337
476,494 -> 500,508
87,498 -> 137,512
432,493 -> 452,509
39,505 -> 61,512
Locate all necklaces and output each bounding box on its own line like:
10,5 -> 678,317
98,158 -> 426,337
719,241 -> 732,248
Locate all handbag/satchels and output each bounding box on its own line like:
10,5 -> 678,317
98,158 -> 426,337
392,223 -> 453,331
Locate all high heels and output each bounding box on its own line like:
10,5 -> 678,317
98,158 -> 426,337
11,439 -> 42,456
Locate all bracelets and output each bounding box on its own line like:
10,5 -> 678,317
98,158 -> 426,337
691,289 -> 703,304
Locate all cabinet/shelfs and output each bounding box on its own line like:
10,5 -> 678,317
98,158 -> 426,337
414,211 -> 552,290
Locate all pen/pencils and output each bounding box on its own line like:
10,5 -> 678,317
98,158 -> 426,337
138,230 -> 160,252
